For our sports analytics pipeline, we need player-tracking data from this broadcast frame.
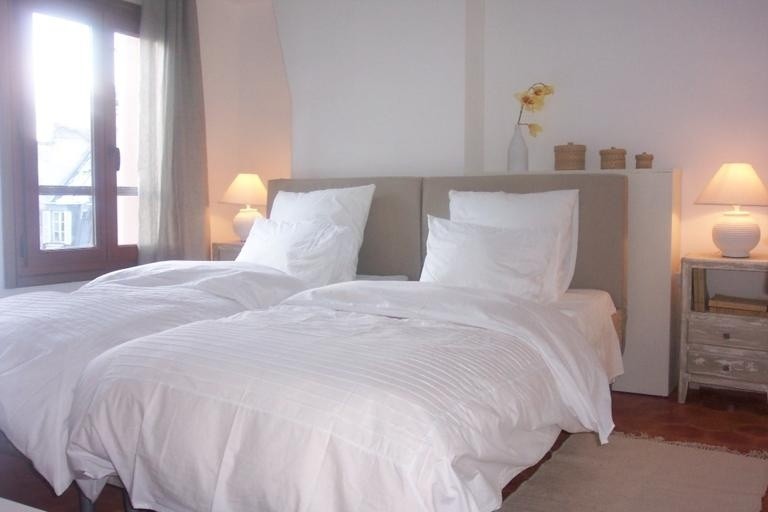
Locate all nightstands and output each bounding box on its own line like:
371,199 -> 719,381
213,244 -> 242,261
676,258 -> 767,403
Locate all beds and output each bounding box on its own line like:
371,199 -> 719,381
1,175 -> 421,511
67,173 -> 628,512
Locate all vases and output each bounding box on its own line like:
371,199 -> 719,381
507,124 -> 527,173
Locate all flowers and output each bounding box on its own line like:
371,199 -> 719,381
513,81 -> 556,136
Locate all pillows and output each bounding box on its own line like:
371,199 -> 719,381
270,183 -> 377,280
234,215 -> 347,288
418,215 -> 562,301
447,188 -> 579,297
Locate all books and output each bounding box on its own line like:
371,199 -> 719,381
708,294 -> 768,318
693,269 -> 706,311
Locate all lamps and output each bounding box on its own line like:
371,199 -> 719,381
222,172 -> 267,242
695,163 -> 766,258
